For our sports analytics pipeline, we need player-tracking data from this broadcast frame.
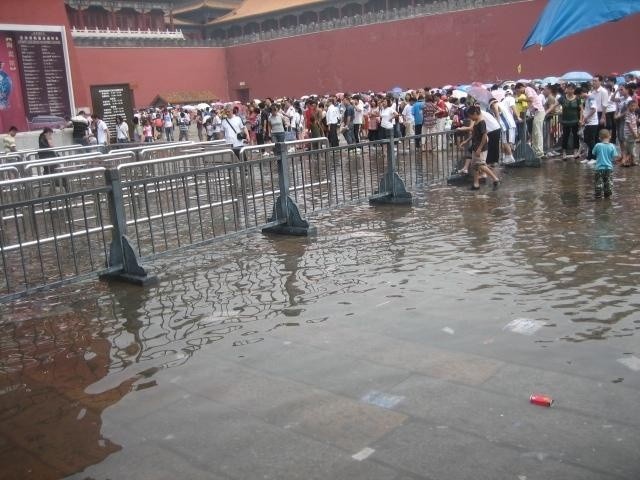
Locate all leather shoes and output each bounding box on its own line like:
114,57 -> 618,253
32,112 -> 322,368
562,158 -> 567,161
575,156 -> 579,159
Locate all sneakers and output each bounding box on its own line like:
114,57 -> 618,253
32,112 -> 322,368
580,158 -> 590,163
471,185 -> 480,190
587,158 -> 597,164
394,140 -> 410,152
547,152 -> 555,157
479,174 -> 487,179
492,179 -> 502,189
251,144 -> 361,156
458,168 -> 468,174
505,157 -> 516,164
553,151 -> 561,155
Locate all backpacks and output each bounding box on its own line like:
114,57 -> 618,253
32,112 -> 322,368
456,106 -> 466,120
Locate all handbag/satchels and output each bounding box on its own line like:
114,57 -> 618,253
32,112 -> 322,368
237,132 -> 246,139
285,118 -> 297,141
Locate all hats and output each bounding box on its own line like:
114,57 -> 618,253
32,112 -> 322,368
489,98 -> 498,108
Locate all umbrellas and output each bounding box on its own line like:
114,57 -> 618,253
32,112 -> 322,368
521,0 -> 640,53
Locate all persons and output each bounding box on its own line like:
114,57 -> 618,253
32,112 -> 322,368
65,71 -> 639,179
39,127 -> 69,194
459,106 -> 502,191
592,128 -> 618,200
3,126 -> 18,153
